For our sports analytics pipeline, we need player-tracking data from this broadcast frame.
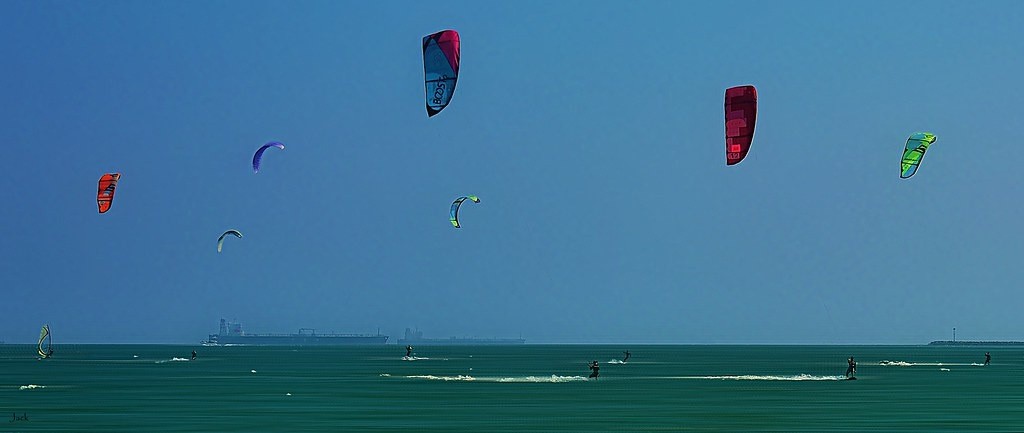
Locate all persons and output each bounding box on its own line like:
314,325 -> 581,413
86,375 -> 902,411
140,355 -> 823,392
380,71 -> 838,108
192,350 -> 197,359
405,345 -> 414,356
846,356 -> 857,377
623,350 -> 631,362
589,361 -> 600,379
985,351 -> 991,364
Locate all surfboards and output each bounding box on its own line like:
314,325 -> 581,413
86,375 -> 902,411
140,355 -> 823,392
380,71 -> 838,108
839,376 -> 858,381
